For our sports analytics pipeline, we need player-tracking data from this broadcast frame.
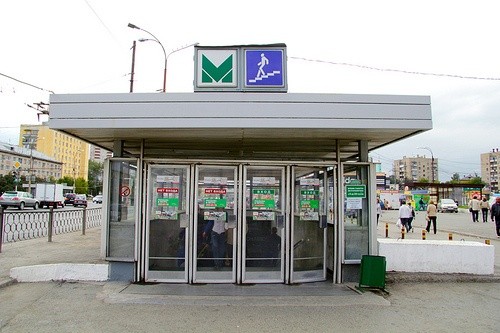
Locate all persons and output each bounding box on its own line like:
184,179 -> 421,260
328,195 -> 500,238
178,203 -> 282,270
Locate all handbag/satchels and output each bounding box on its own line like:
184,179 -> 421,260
412,206 -> 415,217
395,219 -> 401,229
469,207 -> 472,212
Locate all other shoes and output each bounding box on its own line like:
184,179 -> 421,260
409,226 -> 412,230
407,229 -> 409,233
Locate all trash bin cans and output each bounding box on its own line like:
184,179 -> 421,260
355,255 -> 389,294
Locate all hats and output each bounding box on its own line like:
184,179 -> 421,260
496,197 -> 500,202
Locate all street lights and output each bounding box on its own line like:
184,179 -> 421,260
418,147 -> 435,183
128,22 -> 167,92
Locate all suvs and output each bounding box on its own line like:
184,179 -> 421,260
63,193 -> 77,206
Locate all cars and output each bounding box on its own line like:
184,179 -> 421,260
0,191 -> 40,211
73,196 -> 88,207
437,199 -> 459,213
92,195 -> 102,204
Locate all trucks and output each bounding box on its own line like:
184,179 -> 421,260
35,183 -> 75,209
